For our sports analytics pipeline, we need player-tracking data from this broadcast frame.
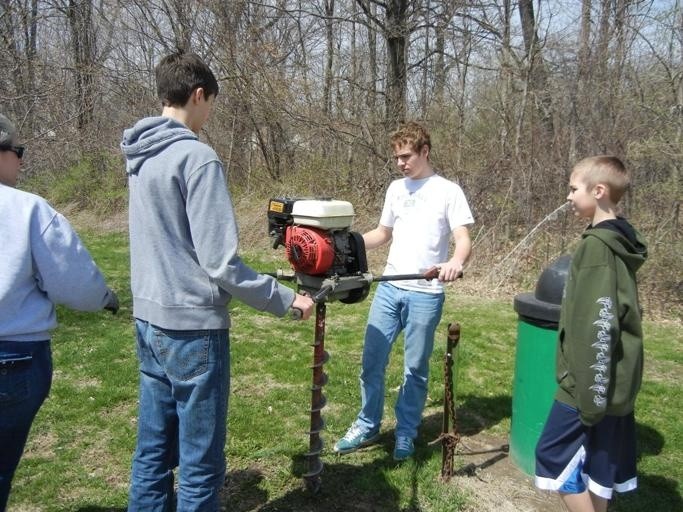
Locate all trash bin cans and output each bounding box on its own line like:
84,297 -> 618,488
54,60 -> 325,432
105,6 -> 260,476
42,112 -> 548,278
508,253 -> 574,481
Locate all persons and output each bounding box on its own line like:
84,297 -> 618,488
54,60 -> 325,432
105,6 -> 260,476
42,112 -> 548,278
334,121 -> 477,461
0,112 -> 119,512
535,156 -> 648,512
119,52 -> 314,512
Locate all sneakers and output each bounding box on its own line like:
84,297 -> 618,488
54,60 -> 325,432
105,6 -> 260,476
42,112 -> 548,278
334,423 -> 379,454
393,436 -> 415,461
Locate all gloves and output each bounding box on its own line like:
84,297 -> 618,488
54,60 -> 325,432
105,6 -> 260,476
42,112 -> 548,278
104,292 -> 119,315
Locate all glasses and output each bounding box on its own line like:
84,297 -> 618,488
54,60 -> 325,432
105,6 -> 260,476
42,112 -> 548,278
0,144 -> 24,158
